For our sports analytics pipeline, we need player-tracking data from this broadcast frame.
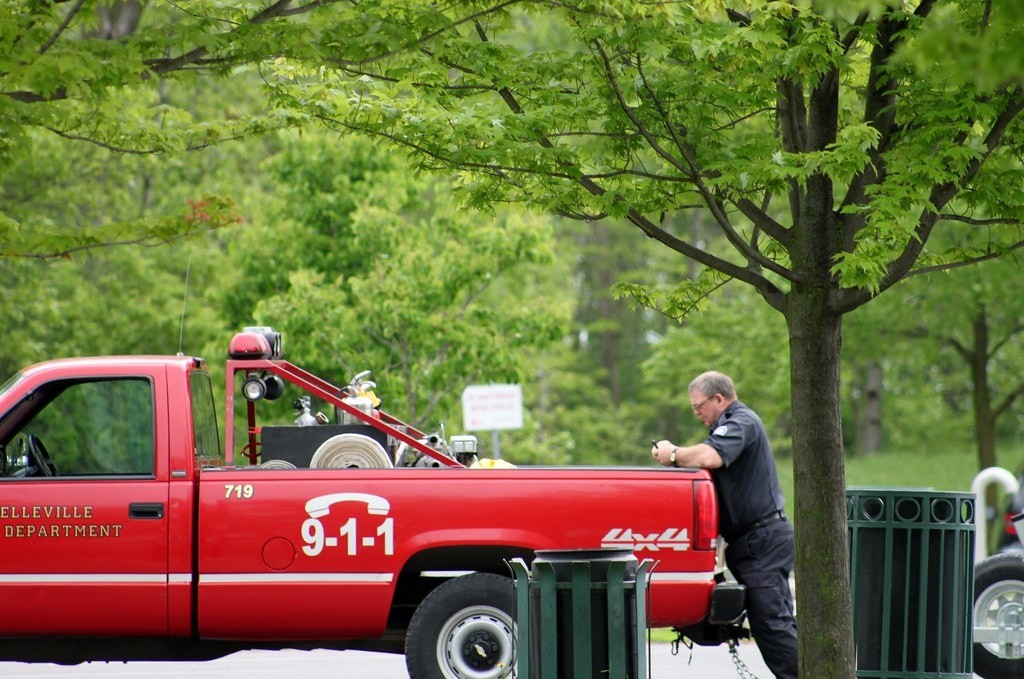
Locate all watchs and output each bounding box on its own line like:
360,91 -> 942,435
671,449 -> 679,468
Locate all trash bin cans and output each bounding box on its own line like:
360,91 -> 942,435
845,486 -> 979,679
502,543 -> 662,679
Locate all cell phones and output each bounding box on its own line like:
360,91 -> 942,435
652,440 -> 658,449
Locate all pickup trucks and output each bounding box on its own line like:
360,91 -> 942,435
0,326 -> 747,679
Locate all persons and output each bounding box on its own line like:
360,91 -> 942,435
652,371 -> 800,679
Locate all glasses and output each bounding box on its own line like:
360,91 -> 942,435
692,395 -> 715,410
